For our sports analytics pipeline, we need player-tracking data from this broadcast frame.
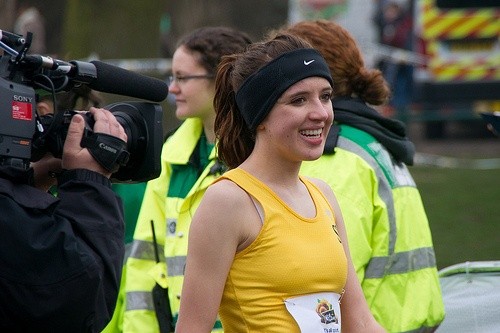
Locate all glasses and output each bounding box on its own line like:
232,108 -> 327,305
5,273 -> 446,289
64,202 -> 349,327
169,75 -> 220,85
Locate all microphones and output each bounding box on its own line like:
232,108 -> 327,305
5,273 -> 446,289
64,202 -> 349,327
38,56 -> 168,102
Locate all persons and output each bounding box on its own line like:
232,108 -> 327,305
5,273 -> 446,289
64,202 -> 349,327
121,27 -> 255,333
287,19 -> 446,333
0,82 -> 128,333
174,32 -> 388,333
